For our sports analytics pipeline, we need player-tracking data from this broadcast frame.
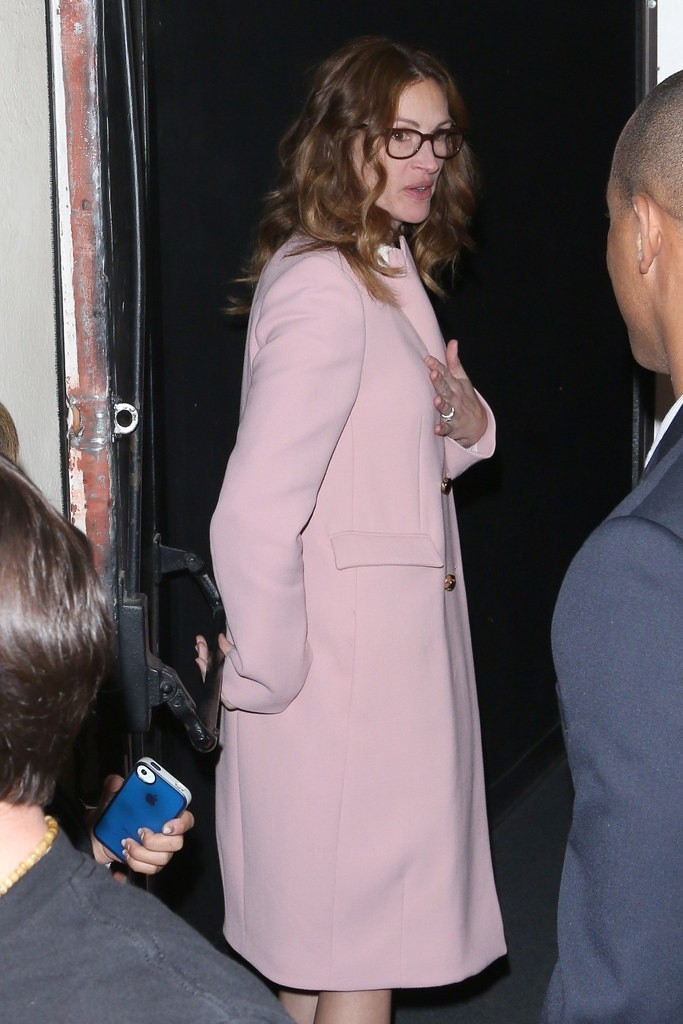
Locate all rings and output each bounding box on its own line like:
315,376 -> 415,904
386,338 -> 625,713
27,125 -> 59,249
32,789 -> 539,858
441,406 -> 455,422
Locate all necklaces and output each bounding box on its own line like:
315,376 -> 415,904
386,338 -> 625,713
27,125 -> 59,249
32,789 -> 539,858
1,816 -> 59,896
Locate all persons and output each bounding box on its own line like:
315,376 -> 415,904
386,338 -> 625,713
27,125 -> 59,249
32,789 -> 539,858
195,35 -> 507,1024
0,403 -> 294,1024
541,70 -> 683,1024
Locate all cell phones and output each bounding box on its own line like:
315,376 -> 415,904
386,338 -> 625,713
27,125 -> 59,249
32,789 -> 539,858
93,757 -> 192,864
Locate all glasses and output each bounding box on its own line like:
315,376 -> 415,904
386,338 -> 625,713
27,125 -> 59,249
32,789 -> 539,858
356,114 -> 466,160
